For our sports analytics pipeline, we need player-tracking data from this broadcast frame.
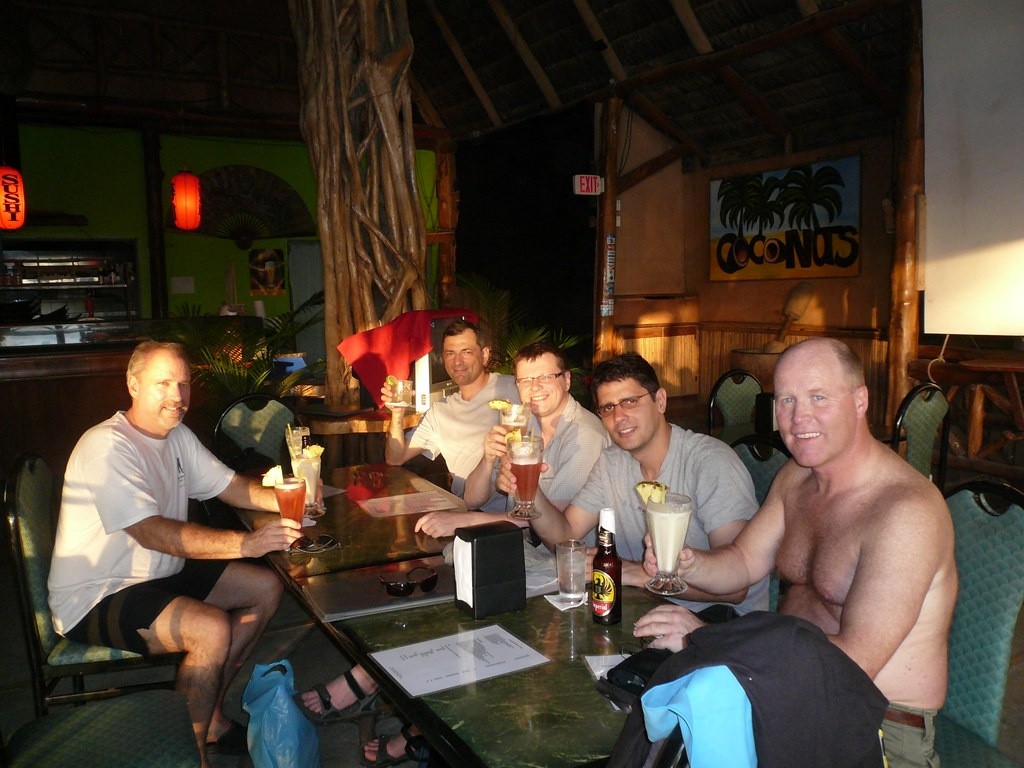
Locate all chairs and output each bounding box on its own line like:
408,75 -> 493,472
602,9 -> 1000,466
0,690 -> 201,768
3,457 -> 194,715
211,392 -> 307,476
706,369 -> 764,437
606,609 -> 891,768
728,435 -> 791,613
892,382 -> 952,485
933,475 -> 1024,767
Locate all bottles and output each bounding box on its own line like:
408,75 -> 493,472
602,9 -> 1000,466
110,264 -> 117,283
592,508 -> 622,624
98,266 -> 104,285
5,272 -> 11,285
15,273 -> 22,286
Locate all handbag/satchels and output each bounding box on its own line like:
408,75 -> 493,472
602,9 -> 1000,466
242,660 -> 321,768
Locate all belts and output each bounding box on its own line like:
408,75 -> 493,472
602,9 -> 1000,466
884,708 -> 926,729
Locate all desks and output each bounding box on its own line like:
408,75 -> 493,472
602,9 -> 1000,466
295,409 -> 419,467
204,466 -> 698,768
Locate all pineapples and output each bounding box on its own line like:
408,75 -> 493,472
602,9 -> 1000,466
262,465 -> 284,487
489,399 -> 511,410
636,482 -> 669,505
306,445 -> 324,459
504,427 -> 522,443
386,375 -> 397,386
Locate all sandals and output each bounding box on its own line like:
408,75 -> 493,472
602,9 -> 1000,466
296,669 -> 384,724
360,723 -> 428,768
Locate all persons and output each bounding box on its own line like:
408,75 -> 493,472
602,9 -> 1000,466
381,321 -> 522,512
632,339 -> 958,767
495,351 -> 770,614
294,342 -> 611,768
47,339 -> 324,767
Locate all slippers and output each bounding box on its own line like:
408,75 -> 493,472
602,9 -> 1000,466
205,719 -> 250,755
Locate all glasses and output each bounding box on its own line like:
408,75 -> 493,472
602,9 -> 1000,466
290,533 -> 341,555
515,371 -> 564,390
596,390 -> 658,419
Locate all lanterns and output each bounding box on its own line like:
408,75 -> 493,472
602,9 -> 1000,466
170,170 -> 203,230
0,161 -> 26,232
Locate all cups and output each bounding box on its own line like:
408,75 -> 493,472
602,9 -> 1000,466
389,380 -> 412,406
639,493 -> 692,596
265,261 -> 274,288
730,348 -> 783,393
507,436 -> 543,521
254,301 -> 264,316
555,539 -> 586,604
285,427 -> 310,460
273,478 -> 306,533
499,404 -> 530,446
290,457 -> 325,518
